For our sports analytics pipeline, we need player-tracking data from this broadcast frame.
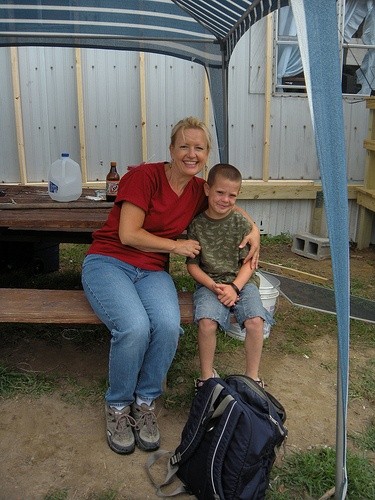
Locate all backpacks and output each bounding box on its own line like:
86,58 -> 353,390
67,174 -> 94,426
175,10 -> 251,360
146,374 -> 288,500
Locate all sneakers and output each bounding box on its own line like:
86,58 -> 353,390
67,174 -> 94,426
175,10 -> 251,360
193,368 -> 220,397
105,401 -> 136,454
130,399 -> 160,452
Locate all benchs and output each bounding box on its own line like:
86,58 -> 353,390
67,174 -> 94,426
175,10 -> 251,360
0,287 -> 239,395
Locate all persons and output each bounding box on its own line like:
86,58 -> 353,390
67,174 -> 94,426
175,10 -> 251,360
81,117 -> 261,456
185,164 -> 267,395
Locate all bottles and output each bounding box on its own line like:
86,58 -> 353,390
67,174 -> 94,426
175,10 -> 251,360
105,162 -> 120,202
47,153 -> 82,202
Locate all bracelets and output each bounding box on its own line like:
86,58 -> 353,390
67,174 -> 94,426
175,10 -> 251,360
229,283 -> 240,295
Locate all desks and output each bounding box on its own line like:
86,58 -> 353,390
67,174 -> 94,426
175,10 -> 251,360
0,183 -> 189,241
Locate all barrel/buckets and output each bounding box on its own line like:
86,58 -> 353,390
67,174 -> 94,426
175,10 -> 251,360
225,272 -> 280,341
